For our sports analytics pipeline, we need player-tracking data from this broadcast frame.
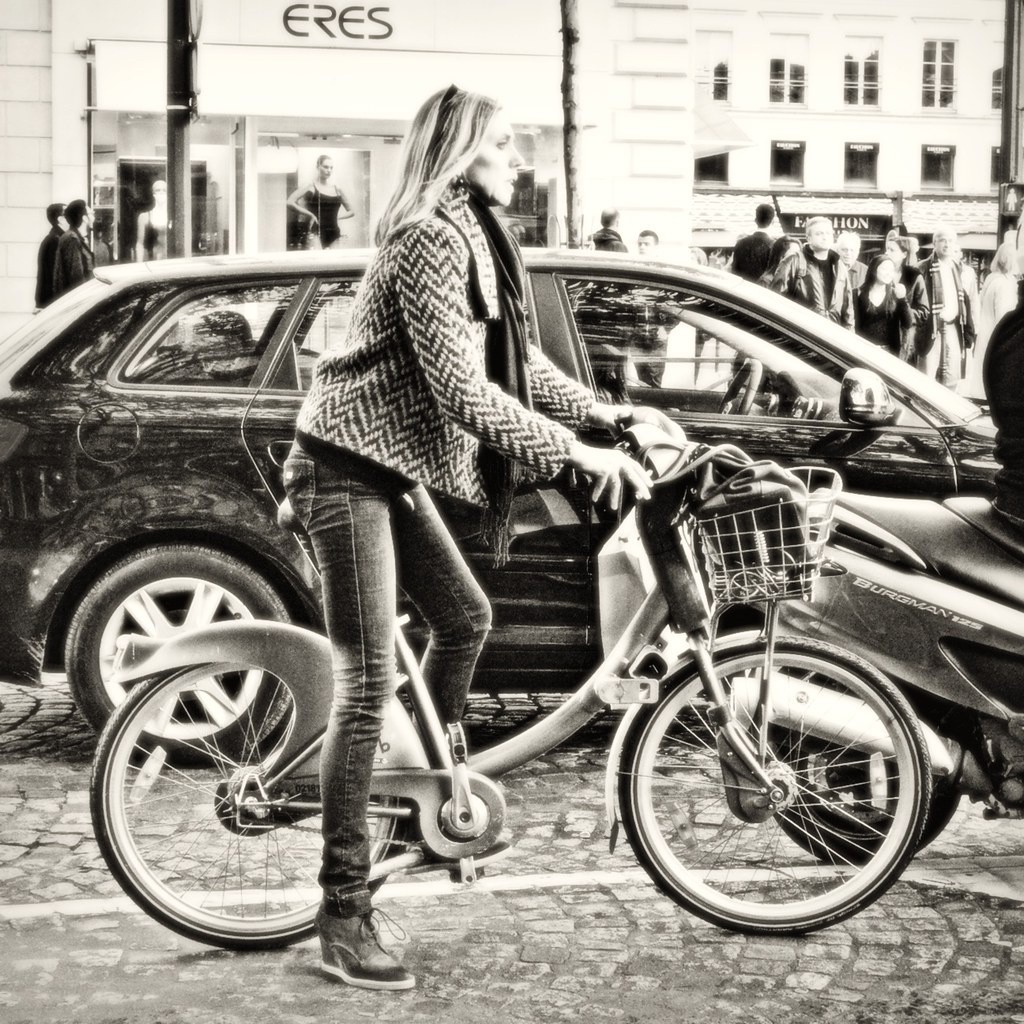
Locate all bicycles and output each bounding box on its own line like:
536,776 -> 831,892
89,409 -> 933,951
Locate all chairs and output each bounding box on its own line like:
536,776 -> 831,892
195,313 -> 258,389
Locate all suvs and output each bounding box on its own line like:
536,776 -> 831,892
1,247 -> 998,767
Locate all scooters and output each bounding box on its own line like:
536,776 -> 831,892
728,474 -> 1024,865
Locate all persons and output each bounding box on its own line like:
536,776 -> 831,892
589,206 -> 659,257
281,85 -> 656,990
287,154 -> 356,249
33,199 -> 96,314
135,180 -> 169,264
734,203 -> 1024,393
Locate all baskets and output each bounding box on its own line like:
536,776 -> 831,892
699,467 -> 841,603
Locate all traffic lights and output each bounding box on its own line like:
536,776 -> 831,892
999,182 -> 1024,217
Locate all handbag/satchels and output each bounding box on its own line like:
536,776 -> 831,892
634,440 -> 807,569
307,232 -> 322,250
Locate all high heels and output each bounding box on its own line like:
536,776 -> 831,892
316,904 -> 418,989
